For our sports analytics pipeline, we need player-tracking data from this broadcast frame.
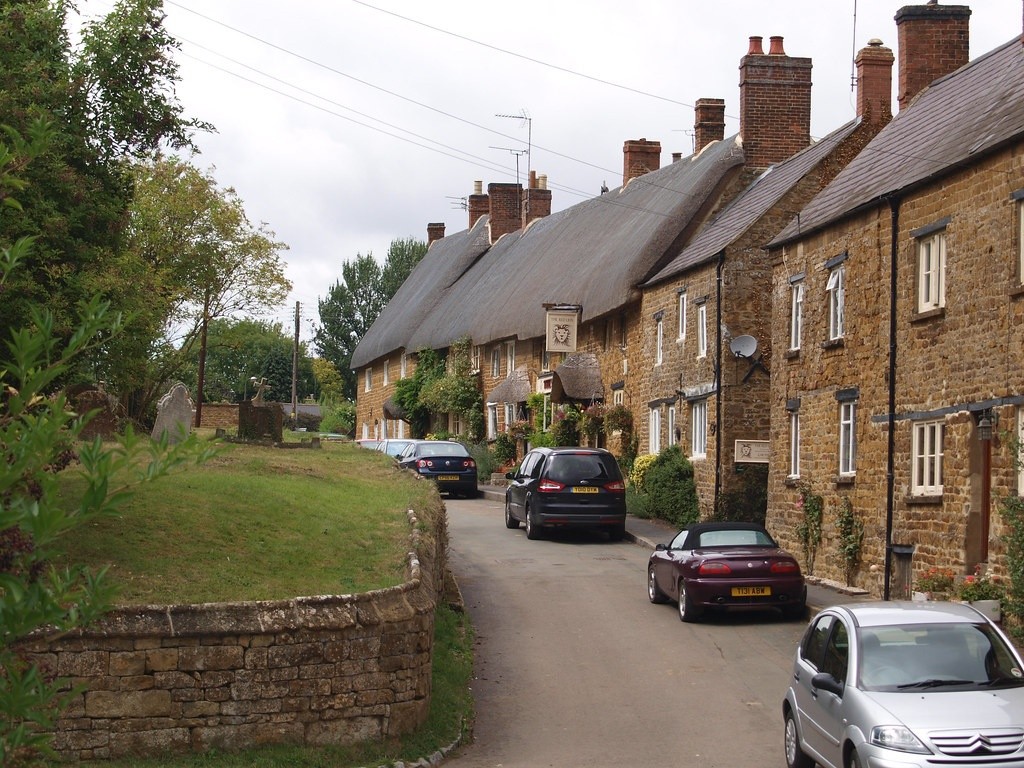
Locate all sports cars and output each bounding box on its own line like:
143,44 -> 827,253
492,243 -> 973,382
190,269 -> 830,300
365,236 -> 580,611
648,522 -> 807,622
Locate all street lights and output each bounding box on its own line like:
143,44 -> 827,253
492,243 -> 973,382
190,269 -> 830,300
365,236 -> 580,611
243,377 -> 257,401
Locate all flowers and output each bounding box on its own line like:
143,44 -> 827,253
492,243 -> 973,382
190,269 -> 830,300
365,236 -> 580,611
950,565 -> 1002,600
506,419 -> 530,429
588,401 -> 608,417
903,567 -> 955,598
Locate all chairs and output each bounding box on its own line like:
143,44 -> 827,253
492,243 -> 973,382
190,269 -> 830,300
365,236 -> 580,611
860,633 -> 881,677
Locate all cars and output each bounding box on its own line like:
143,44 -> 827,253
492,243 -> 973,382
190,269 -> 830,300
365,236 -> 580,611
395,441 -> 477,499
782,603 -> 1024,768
354,439 -> 423,458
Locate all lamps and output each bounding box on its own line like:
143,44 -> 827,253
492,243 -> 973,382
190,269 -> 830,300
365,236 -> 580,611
977,410 -> 999,441
375,419 -> 378,424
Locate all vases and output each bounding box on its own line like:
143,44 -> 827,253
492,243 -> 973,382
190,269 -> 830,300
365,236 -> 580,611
911,590 -> 948,602
510,428 -> 528,438
950,598 -> 1000,622
591,417 -> 604,425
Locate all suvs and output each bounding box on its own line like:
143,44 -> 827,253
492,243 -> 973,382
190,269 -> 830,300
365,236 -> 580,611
505,447 -> 626,540
